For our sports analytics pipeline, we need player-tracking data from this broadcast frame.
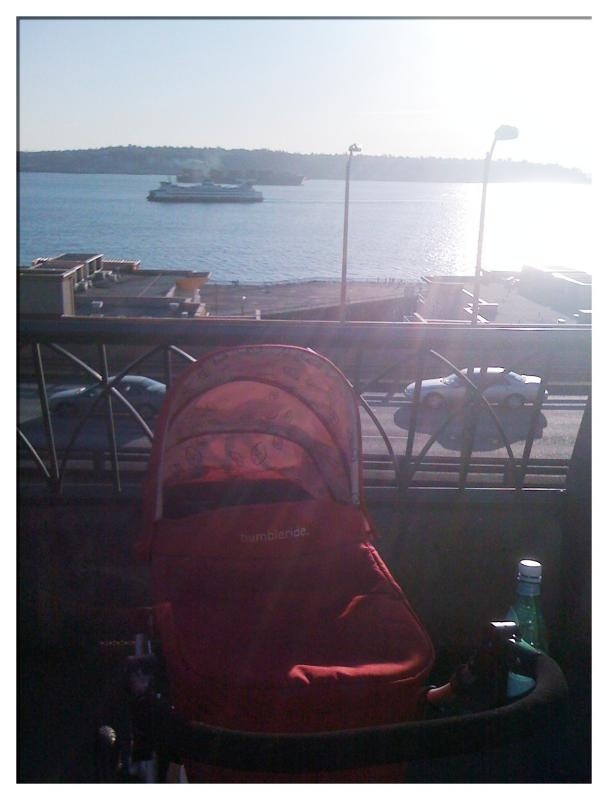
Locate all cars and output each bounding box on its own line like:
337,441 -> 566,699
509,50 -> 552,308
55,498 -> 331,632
403,366 -> 548,410
47,374 -> 169,423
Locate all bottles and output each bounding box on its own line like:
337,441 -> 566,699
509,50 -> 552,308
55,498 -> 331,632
503,559 -> 549,698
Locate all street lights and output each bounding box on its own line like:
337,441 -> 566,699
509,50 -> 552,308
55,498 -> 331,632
340,143 -> 364,321
470,125 -> 519,323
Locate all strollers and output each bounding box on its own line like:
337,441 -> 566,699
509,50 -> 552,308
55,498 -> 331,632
95,343 -> 566,783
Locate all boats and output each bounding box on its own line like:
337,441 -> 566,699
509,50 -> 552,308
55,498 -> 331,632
146,178 -> 265,201
